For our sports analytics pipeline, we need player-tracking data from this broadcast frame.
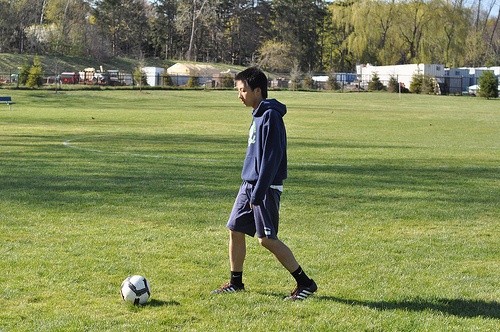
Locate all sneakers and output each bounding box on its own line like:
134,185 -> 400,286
211,282 -> 244,294
283,278 -> 317,302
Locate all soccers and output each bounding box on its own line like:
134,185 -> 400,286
119,274 -> 152,304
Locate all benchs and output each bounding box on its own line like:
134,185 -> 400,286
0,96 -> 16,110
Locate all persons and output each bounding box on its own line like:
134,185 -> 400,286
209,67 -> 318,303
